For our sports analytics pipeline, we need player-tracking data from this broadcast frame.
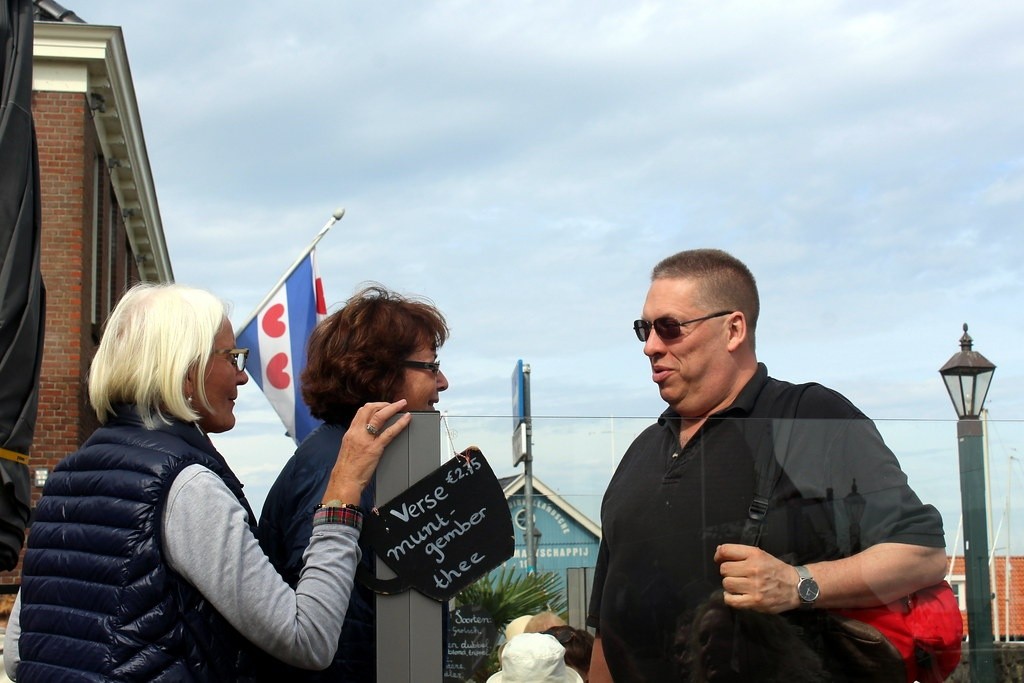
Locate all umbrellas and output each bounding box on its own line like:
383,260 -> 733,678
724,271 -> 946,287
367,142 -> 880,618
0,1 -> 46,572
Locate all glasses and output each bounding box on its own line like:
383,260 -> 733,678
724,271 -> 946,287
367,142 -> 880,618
632,310 -> 734,341
210,348 -> 250,372
400,360 -> 441,376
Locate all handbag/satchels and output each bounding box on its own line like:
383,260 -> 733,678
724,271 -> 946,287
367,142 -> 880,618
727,383 -> 967,683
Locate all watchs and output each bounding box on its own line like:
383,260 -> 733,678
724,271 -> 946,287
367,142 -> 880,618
795,566 -> 820,609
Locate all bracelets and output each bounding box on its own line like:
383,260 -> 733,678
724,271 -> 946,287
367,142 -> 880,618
314,499 -> 365,514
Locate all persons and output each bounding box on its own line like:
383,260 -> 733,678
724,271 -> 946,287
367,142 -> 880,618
587,249 -> 948,683
257,288 -> 450,683
486,615 -> 595,683
0,284 -> 412,683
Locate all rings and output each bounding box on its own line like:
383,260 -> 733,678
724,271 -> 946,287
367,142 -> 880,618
366,424 -> 381,436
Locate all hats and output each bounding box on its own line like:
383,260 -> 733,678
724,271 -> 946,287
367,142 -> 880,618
524,613 -> 566,633
497,614 -> 532,667
486,633 -> 584,683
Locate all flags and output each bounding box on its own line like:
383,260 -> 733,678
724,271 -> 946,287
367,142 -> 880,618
233,246 -> 329,449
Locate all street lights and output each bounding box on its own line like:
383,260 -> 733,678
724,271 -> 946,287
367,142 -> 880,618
940,321 -> 999,683
